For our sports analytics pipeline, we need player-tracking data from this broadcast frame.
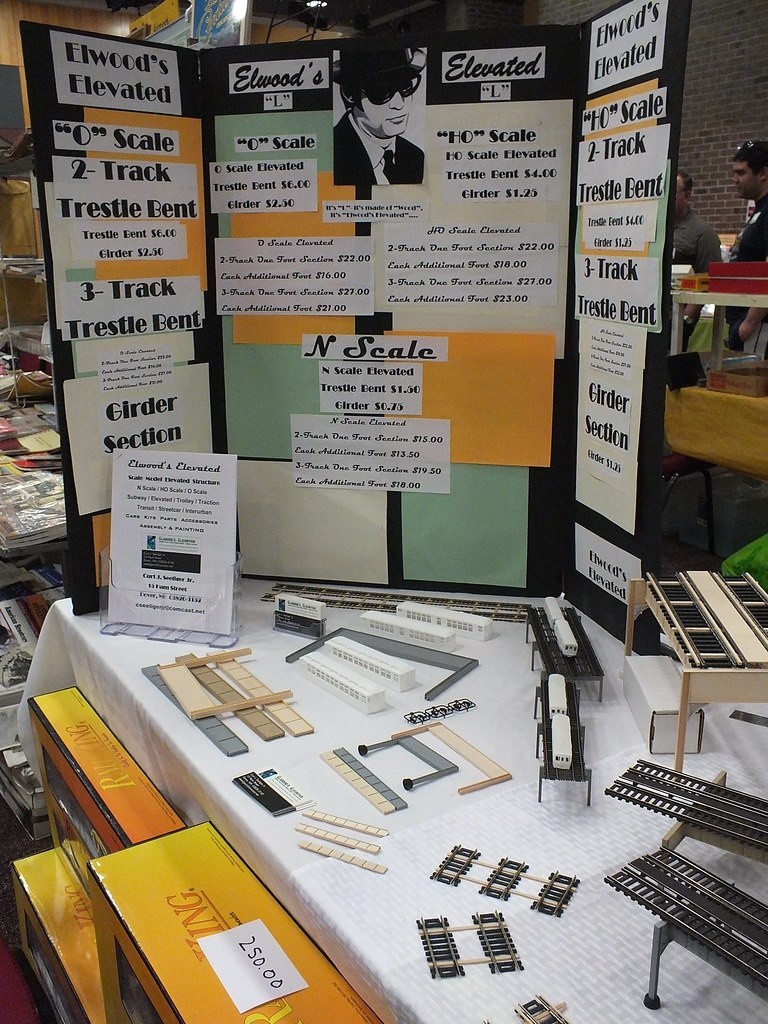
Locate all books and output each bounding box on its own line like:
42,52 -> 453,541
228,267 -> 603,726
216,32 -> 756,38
0,353 -> 68,839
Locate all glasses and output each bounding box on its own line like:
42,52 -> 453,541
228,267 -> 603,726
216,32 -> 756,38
737,139 -> 758,154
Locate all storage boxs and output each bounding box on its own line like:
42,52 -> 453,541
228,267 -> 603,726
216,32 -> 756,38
85,818 -> 384,1024
27,685 -> 188,923
706,366 -> 768,398
669,475 -> 768,559
9,846 -> 105,1024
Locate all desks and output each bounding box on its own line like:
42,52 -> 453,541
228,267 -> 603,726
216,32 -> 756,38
665,385 -> 768,485
15,578 -> 768,1024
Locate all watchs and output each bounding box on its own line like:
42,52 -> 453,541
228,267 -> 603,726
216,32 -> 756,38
684,314 -> 696,325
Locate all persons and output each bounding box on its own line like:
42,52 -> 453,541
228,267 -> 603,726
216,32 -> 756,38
668,171 -> 722,352
723,140 -> 768,359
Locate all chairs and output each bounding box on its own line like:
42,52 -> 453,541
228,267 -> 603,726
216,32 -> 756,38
662,452 -> 716,557
